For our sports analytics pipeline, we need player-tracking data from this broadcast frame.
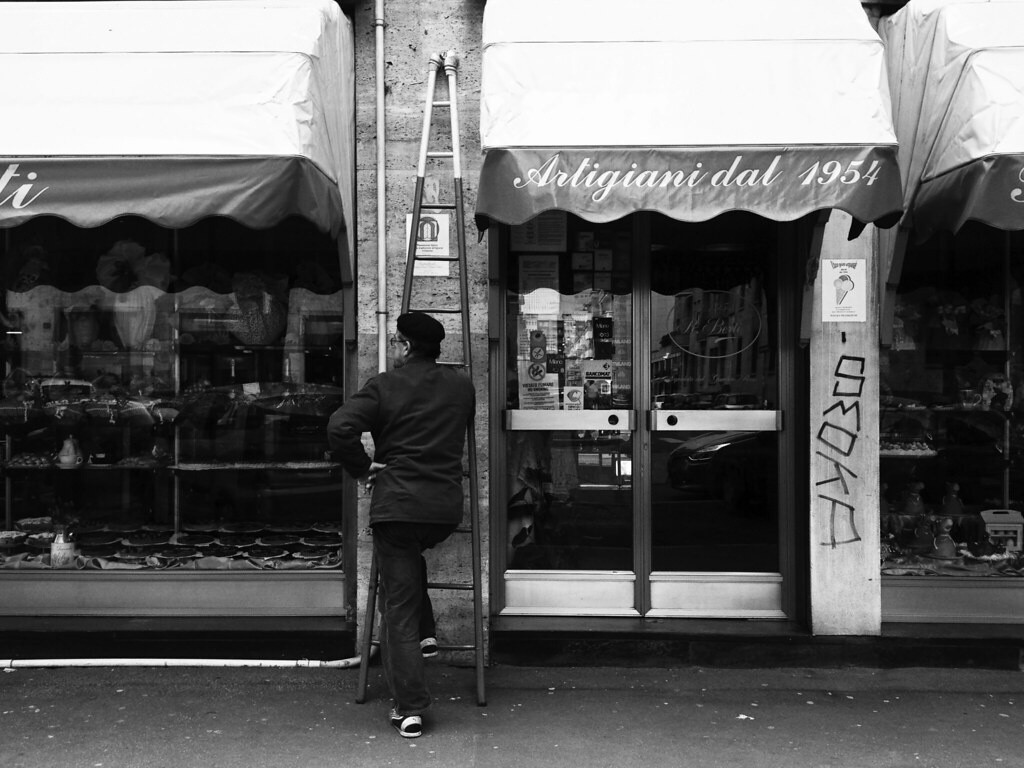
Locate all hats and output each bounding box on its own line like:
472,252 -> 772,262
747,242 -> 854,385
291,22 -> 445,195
397,312 -> 445,343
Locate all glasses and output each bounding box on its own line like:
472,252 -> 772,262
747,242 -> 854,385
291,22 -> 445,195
390,335 -> 412,346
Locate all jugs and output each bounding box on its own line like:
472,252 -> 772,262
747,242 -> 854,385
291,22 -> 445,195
58,433 -> 82,463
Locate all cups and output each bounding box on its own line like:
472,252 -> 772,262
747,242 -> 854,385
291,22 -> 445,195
960,390 -> 982,408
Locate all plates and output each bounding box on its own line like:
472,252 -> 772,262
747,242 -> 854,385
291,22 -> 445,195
0,516 -> 342,564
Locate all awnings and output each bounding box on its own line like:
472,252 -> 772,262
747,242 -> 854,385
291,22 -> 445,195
472,0 -> 903,241
2,0 -> 351,245
877,1 -> 1024,237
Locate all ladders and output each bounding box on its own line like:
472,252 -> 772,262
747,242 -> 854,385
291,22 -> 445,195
357,43 -> 493,705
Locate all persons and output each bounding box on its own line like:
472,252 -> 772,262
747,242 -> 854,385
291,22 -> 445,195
325,310 -> 477,736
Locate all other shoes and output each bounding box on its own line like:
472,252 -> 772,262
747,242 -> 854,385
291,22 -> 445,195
388,705 -> 422,737
420,636 -> 439,658
